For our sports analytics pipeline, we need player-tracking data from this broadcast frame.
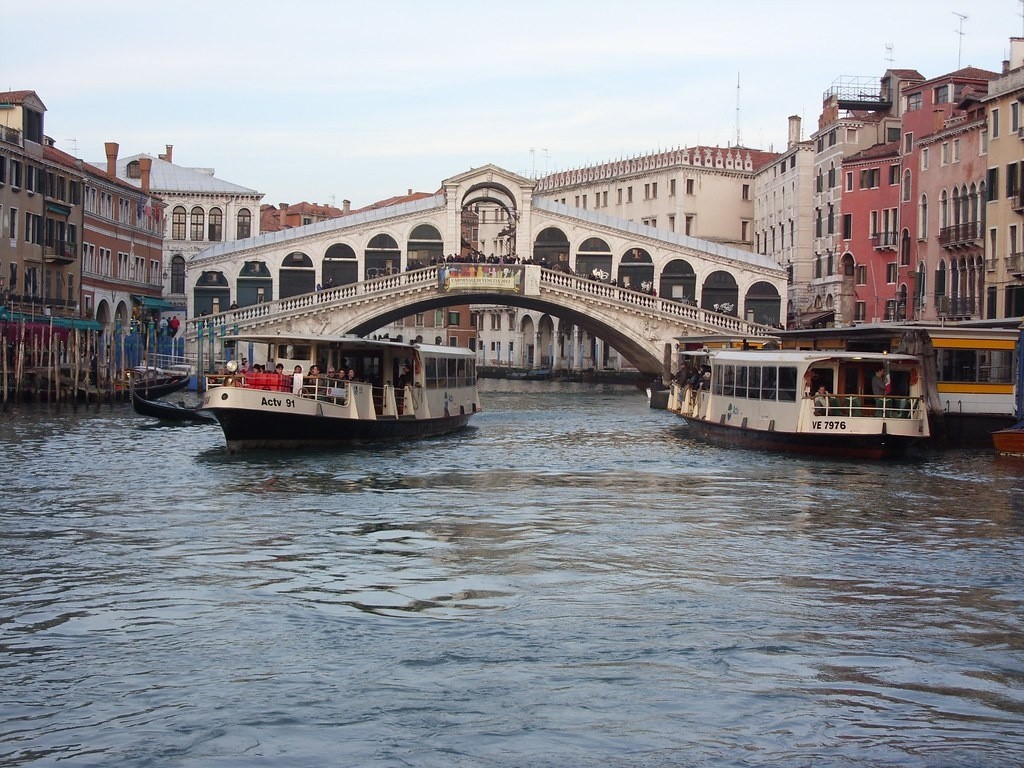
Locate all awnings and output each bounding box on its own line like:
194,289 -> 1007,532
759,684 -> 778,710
132,295 -> 175,311
793,311 -> 834,322
2,312 -> 102,331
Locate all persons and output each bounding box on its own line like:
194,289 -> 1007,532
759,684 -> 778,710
385,250 -> 658,297
317,278 -> 336,300
130,316 -> 180,337
814,385 -> 841,417
690,300 -> 698,307
872,367 -> 886,412
673,361 -> 710,417
230,300 -> 239,316
207,357 -> 413,405
201,310 -> 208,328
769,323 -> 786,330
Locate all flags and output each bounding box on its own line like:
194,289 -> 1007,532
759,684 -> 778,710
136,192 -> 161,223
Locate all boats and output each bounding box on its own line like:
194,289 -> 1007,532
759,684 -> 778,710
202,334 -> 482,454
989,428 -> 1024,453
667,348 -> 929,462
757,326 -> 1020,443
128,376 -> 219,427
